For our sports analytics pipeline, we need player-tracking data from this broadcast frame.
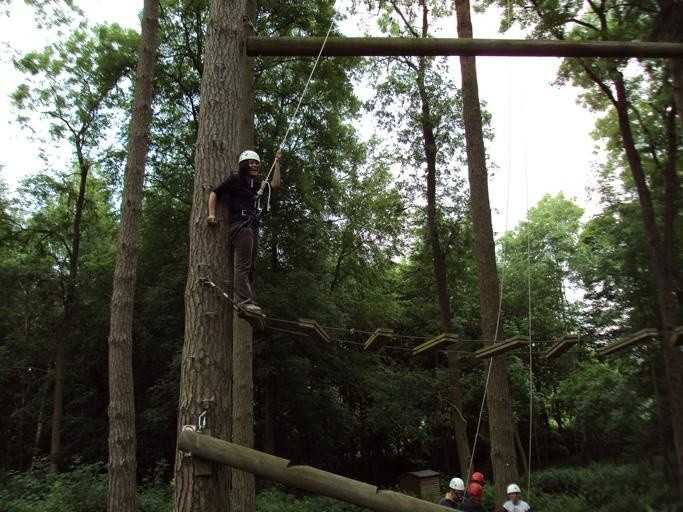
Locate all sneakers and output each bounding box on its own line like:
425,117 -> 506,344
243,305 -> 262,313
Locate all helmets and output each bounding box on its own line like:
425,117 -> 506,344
449,478 -> 465,490
471,472 -> 486,483
468,483 -> 483,497
238,150 -> 261,164
507,484 -> 521,494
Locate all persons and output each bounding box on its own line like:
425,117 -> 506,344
439,472 -> 531,512
206,148 -> 285,318
